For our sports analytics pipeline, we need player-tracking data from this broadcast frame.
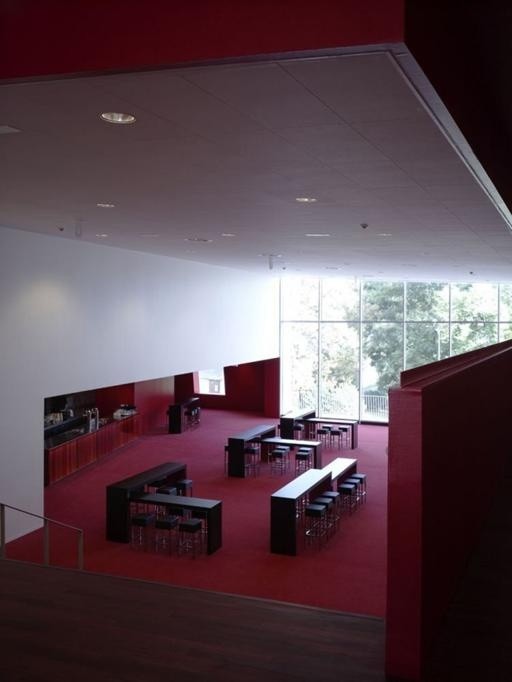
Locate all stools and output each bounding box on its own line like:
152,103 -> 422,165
129,397 -> 368,559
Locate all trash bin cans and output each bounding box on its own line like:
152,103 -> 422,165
208,379 -> 220,393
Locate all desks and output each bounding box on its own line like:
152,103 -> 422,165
104,460 -> 187,545
